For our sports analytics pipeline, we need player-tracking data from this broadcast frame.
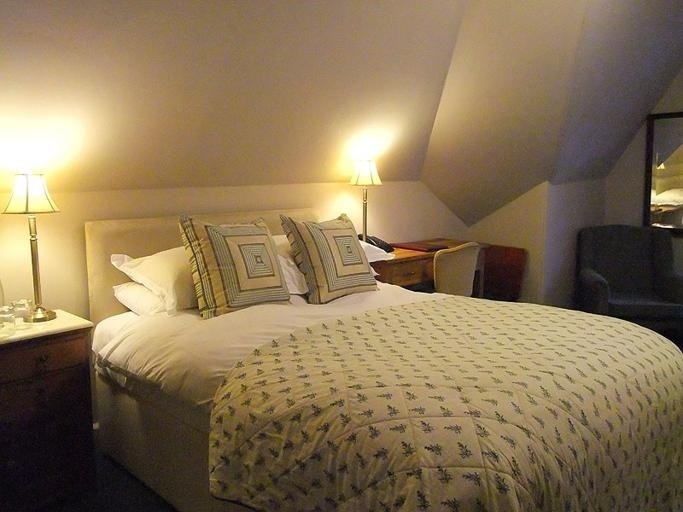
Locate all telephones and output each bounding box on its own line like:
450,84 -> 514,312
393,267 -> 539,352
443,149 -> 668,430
390,241 -> 448,252
358,233 -> 394,253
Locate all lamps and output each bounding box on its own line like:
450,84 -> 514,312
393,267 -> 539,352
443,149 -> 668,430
347,158 -> 384,242
0,171 -> 62,323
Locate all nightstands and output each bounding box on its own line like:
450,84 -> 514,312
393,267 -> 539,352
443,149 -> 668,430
0,306 -> 95,512
369,236 -> 492,300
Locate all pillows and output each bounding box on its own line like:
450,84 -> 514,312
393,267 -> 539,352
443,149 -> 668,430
277,211 -> 381,306
175,211 -> 294,321
112,280 -> 169,317
270,233 -> 397,265
109,244 -> 310,317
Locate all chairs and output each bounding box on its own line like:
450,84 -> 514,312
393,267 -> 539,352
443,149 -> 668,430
424,240 -> 481,299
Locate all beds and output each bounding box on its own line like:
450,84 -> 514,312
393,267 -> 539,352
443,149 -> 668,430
83,204 -> 682,512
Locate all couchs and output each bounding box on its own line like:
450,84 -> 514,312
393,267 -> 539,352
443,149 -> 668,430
573,222 -> 683,355
